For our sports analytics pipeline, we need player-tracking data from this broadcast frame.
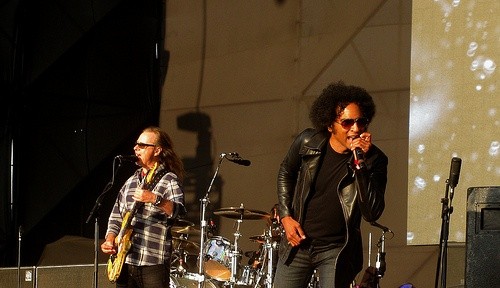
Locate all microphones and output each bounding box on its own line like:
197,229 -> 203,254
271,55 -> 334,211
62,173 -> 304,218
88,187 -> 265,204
116,154 -> 139,164
448,157 -> 461,197
349,137 -> 367,170
224,157 -> 252,167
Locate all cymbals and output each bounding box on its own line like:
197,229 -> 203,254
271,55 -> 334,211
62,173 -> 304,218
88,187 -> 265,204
169,225 -> 200,234
172,237 -> 200,255
212,207 -> 271,221
249,236 -> 281,242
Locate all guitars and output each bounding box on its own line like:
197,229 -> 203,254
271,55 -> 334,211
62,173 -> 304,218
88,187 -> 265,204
106,162 -> 158,283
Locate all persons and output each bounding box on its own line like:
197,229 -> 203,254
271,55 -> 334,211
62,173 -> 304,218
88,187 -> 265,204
101,127 -> 187,288
274,79 -> 389,288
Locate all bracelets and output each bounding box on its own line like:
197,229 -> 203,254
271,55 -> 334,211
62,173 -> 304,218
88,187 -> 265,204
153,195 -> 163,207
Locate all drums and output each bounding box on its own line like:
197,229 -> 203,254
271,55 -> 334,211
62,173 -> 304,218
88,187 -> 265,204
223,266 -> 257,286
197,236 -> 244,282
169,272 -> 220,288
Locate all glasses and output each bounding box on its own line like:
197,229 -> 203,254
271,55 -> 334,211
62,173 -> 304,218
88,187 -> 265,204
336,117 -> 370,131
133,141 -> 158,150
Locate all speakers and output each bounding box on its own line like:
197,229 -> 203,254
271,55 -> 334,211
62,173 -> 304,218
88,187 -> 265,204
462,186 -> 500,288
37,263 -> 116,288
0,266 -> 35,288
